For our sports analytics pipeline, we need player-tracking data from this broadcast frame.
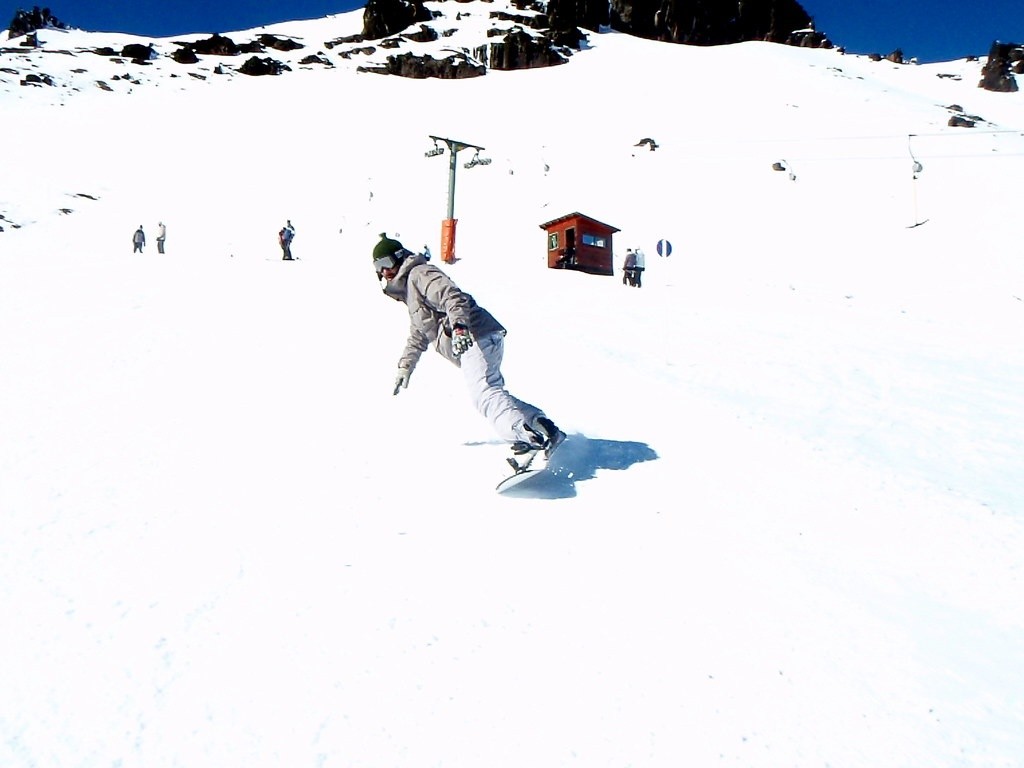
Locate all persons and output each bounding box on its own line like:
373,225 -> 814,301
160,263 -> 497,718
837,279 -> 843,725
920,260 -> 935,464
622,248 -> 636,286
133,225 -> 146,254
279,220 -> 295,260
633,249 -> 645,288
373,232 -> 561,470
156,221 -> 166,253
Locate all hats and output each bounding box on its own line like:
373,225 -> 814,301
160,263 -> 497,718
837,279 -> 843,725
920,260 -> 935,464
370,232 -> 404,281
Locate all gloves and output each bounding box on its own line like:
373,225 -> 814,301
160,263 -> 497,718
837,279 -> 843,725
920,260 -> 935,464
451,327 -> 473,360
392,368 -> 411,396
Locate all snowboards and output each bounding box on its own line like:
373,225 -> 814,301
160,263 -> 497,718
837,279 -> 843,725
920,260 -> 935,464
494,428 -> 568,494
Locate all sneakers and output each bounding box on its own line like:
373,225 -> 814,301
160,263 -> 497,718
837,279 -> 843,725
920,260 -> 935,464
511,418 -> 559,455
